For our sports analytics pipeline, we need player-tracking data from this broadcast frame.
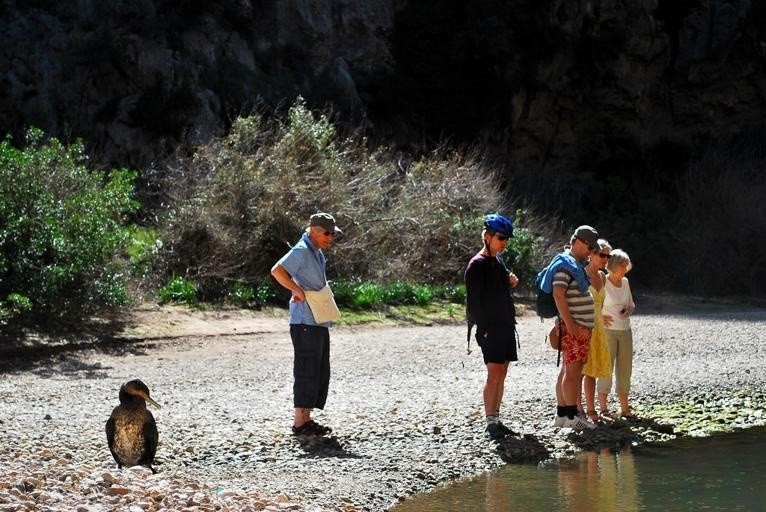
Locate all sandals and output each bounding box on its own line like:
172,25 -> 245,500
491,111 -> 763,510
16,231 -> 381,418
586,410 -> 642,423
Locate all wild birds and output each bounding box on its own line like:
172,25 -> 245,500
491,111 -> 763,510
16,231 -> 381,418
103,377 -> 164,474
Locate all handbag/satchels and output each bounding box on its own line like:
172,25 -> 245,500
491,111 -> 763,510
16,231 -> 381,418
304,281 -> 341,324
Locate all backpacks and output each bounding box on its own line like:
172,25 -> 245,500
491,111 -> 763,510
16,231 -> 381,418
535,265 -> 574,318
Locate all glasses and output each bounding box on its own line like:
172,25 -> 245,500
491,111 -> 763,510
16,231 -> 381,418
320,230 -> 335,236
594,251 -> 611,258
575,235 -> 594,250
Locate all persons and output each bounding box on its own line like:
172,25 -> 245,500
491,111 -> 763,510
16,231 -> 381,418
583,238 -> 611,422
552,224 -> 598,428
462,212 -> 521,438
600,247 -> 634,420
267,212 -> 343,433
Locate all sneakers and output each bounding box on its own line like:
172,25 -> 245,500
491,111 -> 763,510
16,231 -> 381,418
482,421 -> 504,439
290,420 -> 332,439
497,420 -> 519,437
564,416 -> 597,430
554,416 -> 566,427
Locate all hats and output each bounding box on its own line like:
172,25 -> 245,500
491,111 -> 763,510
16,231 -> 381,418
575,224 -> 600,249
309,213 -> 342,235
